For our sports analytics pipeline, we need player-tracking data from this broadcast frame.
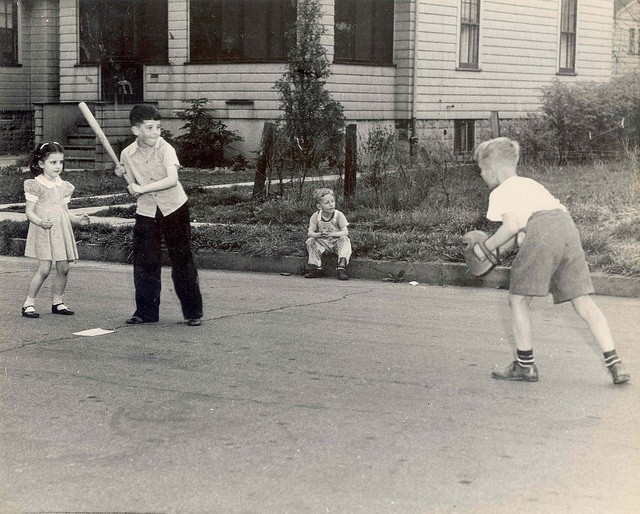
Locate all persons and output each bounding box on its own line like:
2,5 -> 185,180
460,137 -> 631,385
304,187 -> 352,280
22,141 -> 90,318
114,105 -> 204,326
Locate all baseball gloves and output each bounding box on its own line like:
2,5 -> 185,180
461,230 -> 503,278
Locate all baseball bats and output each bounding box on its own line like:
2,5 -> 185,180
77,100 -> 140,198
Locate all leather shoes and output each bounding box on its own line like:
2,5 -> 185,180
22,306 -> 39,319
52,303 -> 75,316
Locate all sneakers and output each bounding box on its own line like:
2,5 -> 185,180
337,269 -> 347,280
610,363 -> 631,384
492,361 -> 539,383
304,270 -> 324,278
125,316 -> 159,325
185,319 -> 202,327
